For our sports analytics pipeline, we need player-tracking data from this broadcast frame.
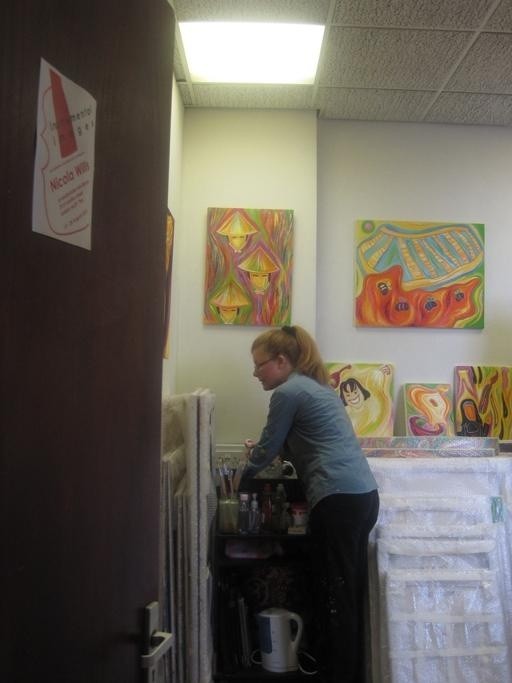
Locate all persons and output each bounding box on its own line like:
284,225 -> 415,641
337,378 -> 371,408
236,326 -> 379,683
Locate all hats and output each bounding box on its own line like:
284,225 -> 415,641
217,211 -> 257,234
238,247 -> 279,273
209,279 -> 252,306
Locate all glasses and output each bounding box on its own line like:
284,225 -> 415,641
254,355 -> 277,370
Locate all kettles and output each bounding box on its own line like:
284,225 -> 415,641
257,608 -> 303,672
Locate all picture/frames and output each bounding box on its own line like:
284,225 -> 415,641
377,521 -> 512,626
455,366 -> 512,440
369,492 -> 491,683
202,207 -> 294,327
404,383 -> 454,436
365,456 -> 512,570
163,207 -> 174,359
322,362 -> 395,437
352,218 -> 485,329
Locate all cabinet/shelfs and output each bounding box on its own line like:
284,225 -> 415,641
208,476 -> 318,683
